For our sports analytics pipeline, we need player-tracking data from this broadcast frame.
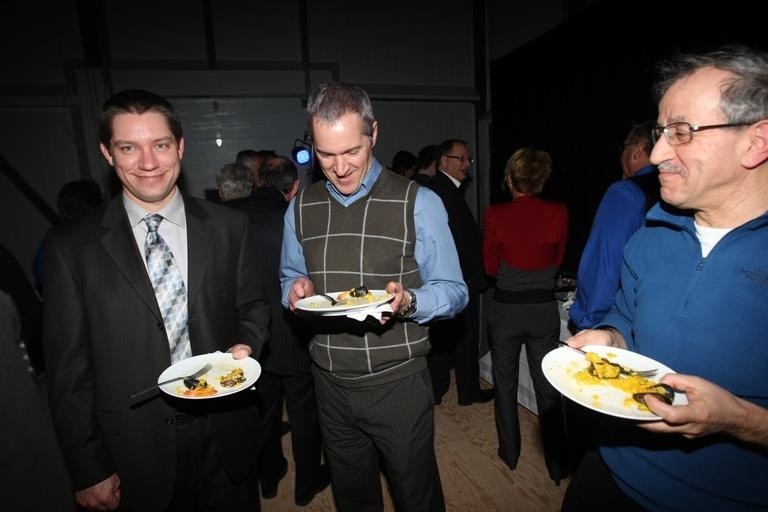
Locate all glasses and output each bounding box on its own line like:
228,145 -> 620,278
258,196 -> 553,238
443,154 -> 475,165
650,119 -> 754,148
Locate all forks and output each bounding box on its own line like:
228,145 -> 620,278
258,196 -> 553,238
128,363 -> 216,399
311,290 -> 349,308
556,338 -> 660,377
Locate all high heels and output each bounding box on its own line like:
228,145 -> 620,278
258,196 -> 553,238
547,463 -> 570,487
498,445 -> 521,471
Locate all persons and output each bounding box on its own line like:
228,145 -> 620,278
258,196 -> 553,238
33,83 -> 496,511
483,149 -> 569,485
560,46 -> 768,512
569,122 -> 660,334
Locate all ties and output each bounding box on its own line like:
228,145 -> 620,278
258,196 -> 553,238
143,213 -> 193,366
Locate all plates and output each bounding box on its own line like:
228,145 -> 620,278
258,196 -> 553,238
294,289 -> 394,318
541,343 -> 692,422
157,349 -> 262,401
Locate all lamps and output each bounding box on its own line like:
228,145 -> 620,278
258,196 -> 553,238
292,138 -> 313,166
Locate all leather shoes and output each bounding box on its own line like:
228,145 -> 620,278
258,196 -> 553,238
294,463 -> 331,507
457,387 -> 495,407
261,457 -> 288,499
